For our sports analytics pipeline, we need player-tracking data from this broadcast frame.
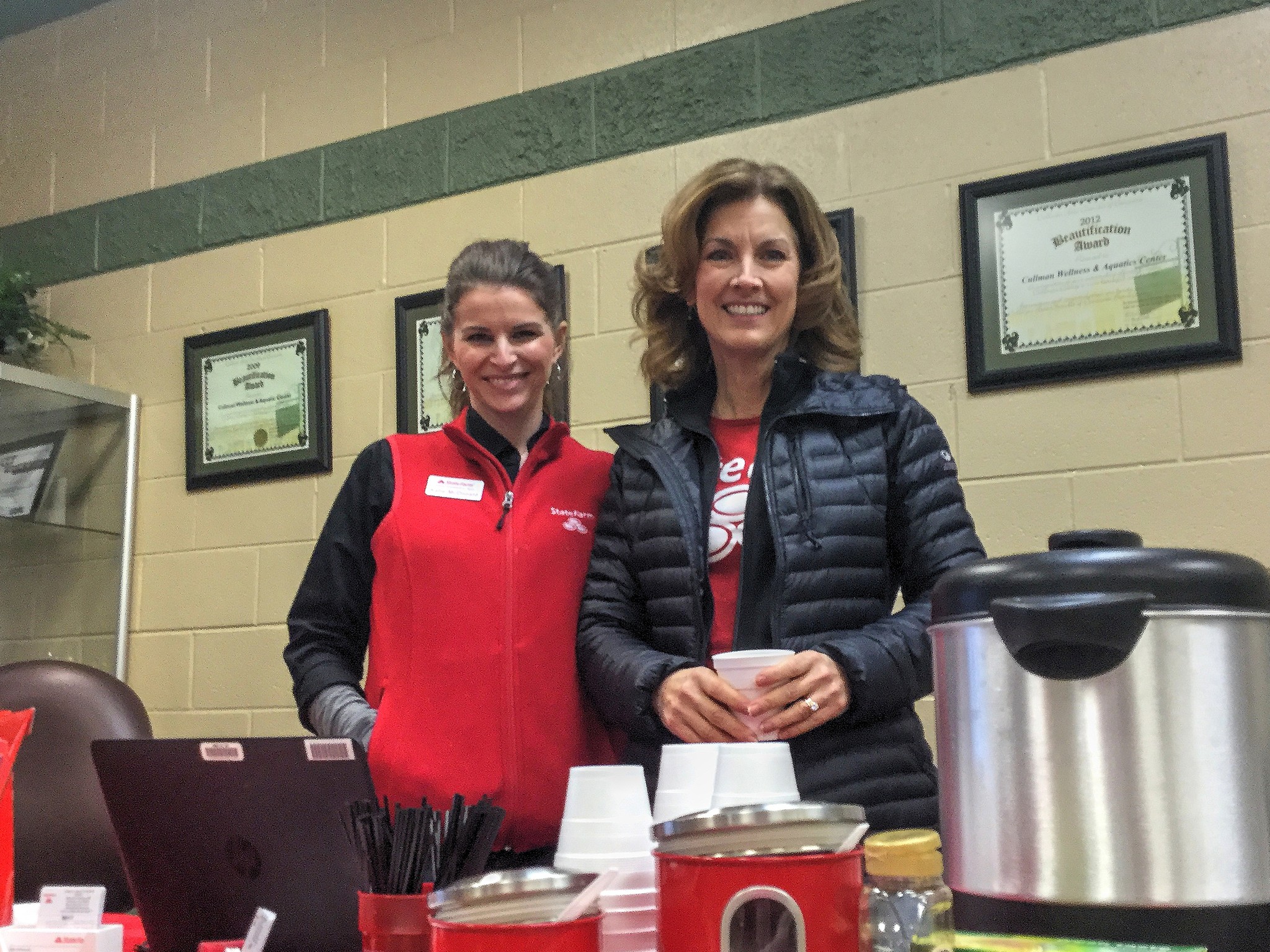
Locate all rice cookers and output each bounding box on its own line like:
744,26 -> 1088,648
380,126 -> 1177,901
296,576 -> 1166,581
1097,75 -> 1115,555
919,528 -> 1270,952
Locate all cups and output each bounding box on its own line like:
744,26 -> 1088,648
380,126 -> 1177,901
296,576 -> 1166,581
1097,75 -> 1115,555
707,646 -> 798,745
352,886 -> 601,952
546,739 -> 802,951
652,847 -> 865,952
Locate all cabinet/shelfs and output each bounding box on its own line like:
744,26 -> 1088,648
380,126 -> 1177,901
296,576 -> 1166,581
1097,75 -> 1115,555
0,359 -> 144,538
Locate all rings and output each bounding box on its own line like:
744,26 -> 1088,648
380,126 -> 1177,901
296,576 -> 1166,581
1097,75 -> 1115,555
805,698 -> 820,713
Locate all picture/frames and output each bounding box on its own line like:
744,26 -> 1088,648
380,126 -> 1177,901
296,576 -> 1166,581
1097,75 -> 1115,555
642,206 -> 858,422
957,129 -> 1243,394
0,428 -> 67,523
181,306 -> 331,492
394,263 -> 572,435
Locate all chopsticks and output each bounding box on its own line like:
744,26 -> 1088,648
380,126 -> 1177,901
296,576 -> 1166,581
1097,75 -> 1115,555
332,789 -> 503,895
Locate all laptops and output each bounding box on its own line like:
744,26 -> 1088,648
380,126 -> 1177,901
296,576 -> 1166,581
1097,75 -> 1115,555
89,737 -> 386,952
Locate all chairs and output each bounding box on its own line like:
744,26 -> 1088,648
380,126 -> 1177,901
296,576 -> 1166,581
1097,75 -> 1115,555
1,657 -> 153,916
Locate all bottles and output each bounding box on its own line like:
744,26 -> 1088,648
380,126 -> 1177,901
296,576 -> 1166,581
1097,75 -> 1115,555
860,831 -> 954,952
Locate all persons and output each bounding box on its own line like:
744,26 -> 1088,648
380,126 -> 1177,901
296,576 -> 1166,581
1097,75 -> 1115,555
572,158 -> 988,845
280,239 -> 619,883
